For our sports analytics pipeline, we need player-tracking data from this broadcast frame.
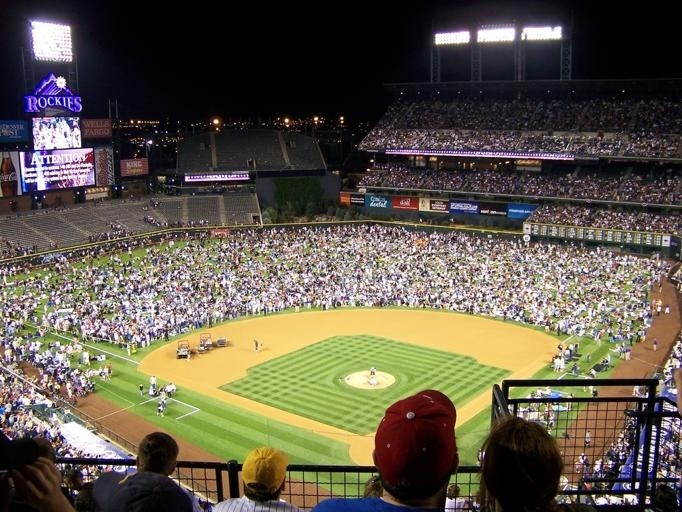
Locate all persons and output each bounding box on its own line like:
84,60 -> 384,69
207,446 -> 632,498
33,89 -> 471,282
446,338 -> 682,512
311,390 -> 459,512
210,446 -> 304,512
370,366 -> 376,376
1,86 -> 446,351
446,85 -> 681,338
116,431 -> 205,512
2,351 -> 176,511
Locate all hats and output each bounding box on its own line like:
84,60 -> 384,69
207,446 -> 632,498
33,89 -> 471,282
242,446 -> 289,493
91,471 -> 193,512
372,389 -> 456,484
